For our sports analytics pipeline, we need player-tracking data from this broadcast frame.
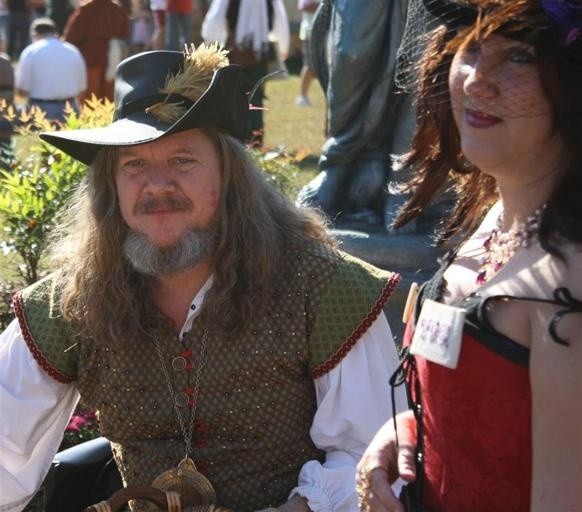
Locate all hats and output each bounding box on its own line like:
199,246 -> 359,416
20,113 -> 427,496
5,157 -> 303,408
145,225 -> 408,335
37,49 -> 258,166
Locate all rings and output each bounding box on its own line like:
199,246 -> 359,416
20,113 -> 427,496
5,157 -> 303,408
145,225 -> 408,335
358,460 -> 388,511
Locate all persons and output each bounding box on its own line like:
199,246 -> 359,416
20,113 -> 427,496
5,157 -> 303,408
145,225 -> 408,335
354,0 -> 581,512
295,0 -> 323,106
2,41 -> 426,511
0,0 -> 291,150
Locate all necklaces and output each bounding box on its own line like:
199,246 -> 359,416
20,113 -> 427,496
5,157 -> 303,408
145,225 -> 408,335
470,197 -> 549,295
145,330 -> 220,511
